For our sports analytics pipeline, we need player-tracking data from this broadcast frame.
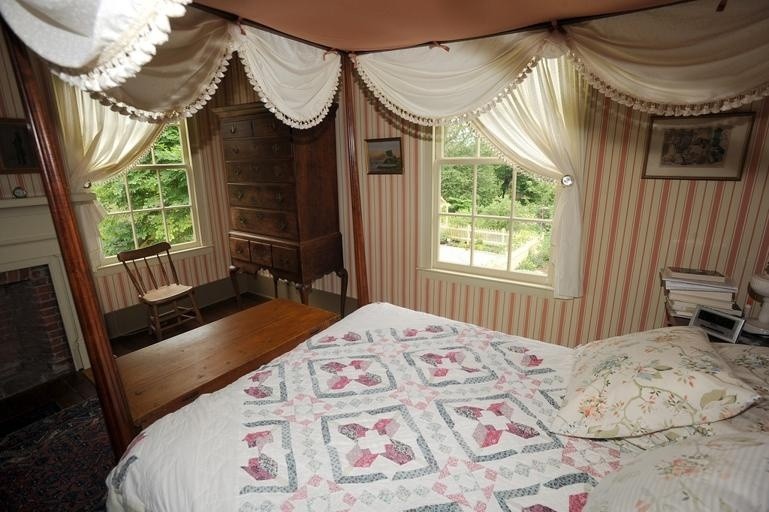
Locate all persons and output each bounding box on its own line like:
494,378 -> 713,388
687,143 -> 706,156
663,134 -> 681,162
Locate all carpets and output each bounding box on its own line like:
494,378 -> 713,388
1,394 -> 118,512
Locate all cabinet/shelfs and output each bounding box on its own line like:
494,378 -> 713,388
210,101 -> 348,318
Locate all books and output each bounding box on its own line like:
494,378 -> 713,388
658,265 -> 743,320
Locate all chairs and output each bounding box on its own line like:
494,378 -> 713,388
118,242 -> 205,341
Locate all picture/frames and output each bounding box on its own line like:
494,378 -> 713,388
363,137 -> 405,175
639,111 -> 756,181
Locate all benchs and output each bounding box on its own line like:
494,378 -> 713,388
110,298 -> 341,434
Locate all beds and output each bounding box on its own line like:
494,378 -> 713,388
105,302 -> 769,512
